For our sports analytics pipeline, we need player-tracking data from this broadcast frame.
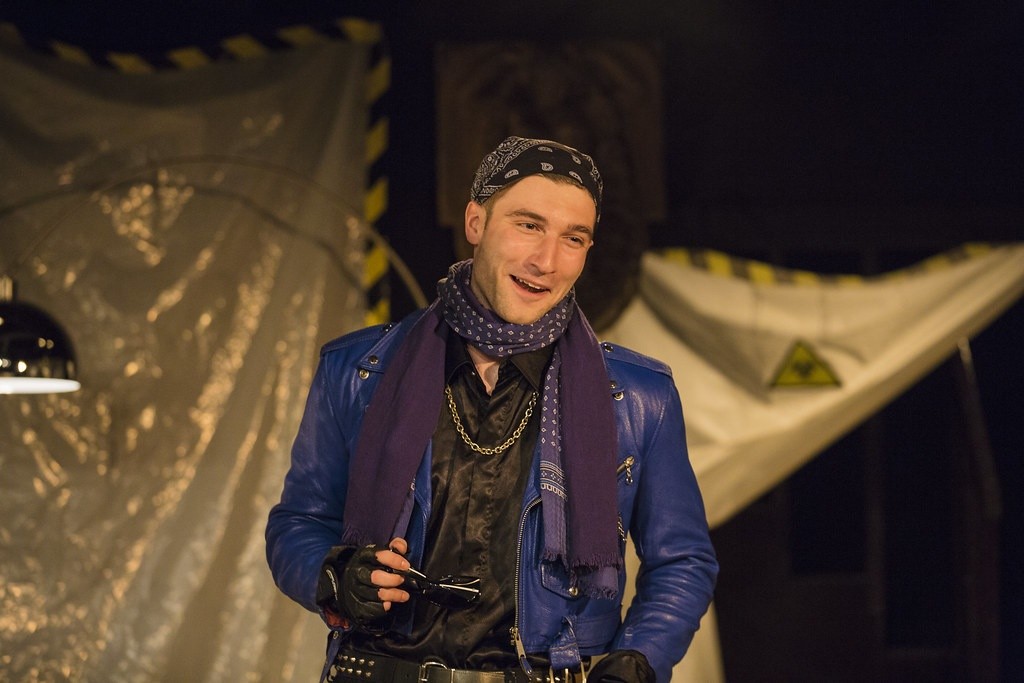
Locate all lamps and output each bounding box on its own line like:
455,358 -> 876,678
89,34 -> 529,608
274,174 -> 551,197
0,154 -> 428,395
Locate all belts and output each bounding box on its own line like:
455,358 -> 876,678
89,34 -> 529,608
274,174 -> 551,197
335,650 -> 576,683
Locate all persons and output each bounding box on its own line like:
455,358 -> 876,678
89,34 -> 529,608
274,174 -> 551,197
264,136 -> 720,683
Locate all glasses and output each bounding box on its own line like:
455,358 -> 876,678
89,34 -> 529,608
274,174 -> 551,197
387,547 -> 481,611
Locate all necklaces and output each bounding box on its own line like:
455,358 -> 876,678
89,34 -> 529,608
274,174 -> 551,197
442,377 -> 541,456
